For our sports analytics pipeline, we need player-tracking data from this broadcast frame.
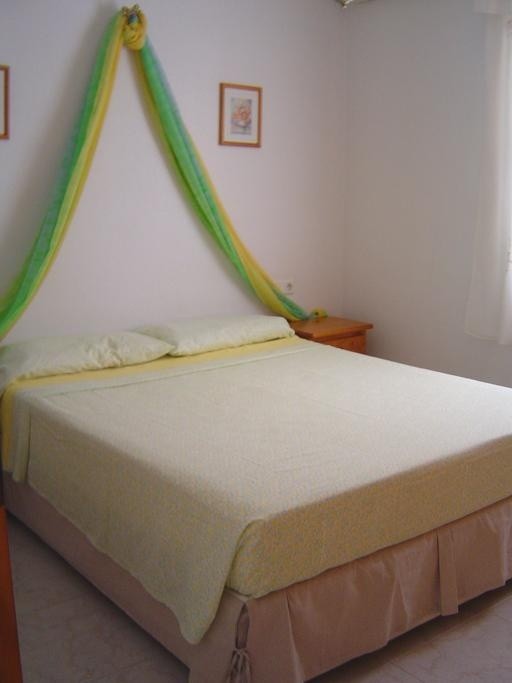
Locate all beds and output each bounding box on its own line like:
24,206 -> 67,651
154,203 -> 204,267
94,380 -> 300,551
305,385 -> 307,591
0,334 -> 512,683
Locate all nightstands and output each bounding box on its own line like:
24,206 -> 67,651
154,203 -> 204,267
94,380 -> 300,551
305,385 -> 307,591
288,315 -> 374,355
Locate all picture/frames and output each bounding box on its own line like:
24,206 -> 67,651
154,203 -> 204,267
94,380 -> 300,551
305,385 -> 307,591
218,81 -> 263,148
0,63 -> 11,140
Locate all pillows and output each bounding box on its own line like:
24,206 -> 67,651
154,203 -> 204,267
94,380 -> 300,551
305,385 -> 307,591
1,331 -> 177,381
138,313 -> 294,358
